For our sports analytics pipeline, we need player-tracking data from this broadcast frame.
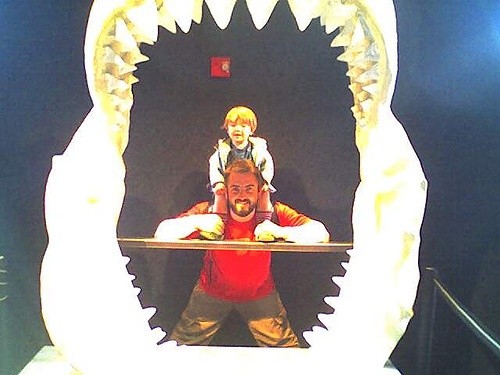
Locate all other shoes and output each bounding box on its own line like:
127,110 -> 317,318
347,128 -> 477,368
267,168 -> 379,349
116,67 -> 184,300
254,235 -> 275,242
200,231 -> 224,241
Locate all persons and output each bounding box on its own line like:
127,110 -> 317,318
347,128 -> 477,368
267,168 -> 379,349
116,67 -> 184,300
153,157 -> 330,349
199,106 -> 277,243
